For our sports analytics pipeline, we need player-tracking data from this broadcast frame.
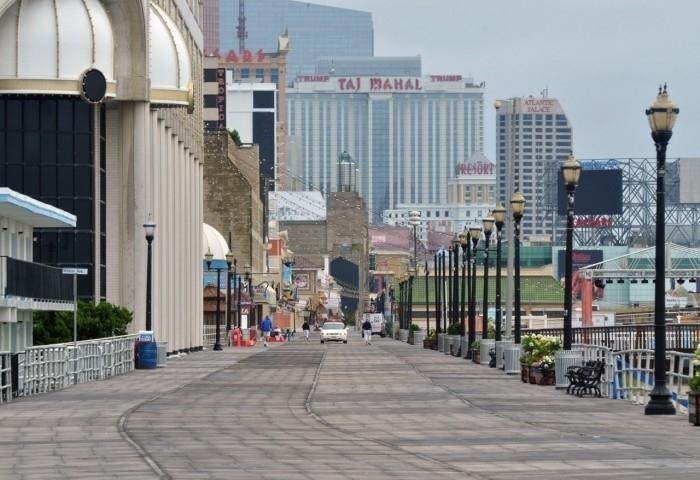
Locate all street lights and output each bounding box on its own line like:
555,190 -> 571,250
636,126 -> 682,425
230,263 -> 252,328
555,148 -> 583,352
406,267 -> 418,328
377,273 -> 408,328
508,189 -> 530,344
491,198 -> 507,344
407,210 -> 423,266
202,246 -> 235,352
140,211 -> 158,332
642,80 -> 681,416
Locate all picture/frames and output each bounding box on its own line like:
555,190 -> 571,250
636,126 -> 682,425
424,340 -> 436,349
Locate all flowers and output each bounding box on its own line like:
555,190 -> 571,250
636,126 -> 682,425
471,340 -> 480,350
688,343 -> 700,391
520,333 -> 560,369
425,329 -> 436,340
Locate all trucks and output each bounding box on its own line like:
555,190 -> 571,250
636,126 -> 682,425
360,311 -> 388,338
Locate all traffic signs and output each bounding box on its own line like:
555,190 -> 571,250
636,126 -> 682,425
62,269 -> 89,276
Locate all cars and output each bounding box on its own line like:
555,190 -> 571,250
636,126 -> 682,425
449,214 -> 495,342
317,321 -> 350,345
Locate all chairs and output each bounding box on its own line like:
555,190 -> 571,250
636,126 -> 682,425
489,348 -> 496,367
564,360 -> 605,397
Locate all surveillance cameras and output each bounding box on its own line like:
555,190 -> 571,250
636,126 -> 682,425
494,99 -> 501,110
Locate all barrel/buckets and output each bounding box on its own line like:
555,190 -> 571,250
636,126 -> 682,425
250,329 -> 257,341
136,341 -> 158,370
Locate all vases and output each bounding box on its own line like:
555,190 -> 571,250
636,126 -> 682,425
520,364 -> 556,385
686,390 -> 700,427
470,349 -> 480,364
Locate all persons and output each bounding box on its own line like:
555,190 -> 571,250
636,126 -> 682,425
261,314 -> 274,346
362,318 -> 372,346
301,320 -> 310,342
